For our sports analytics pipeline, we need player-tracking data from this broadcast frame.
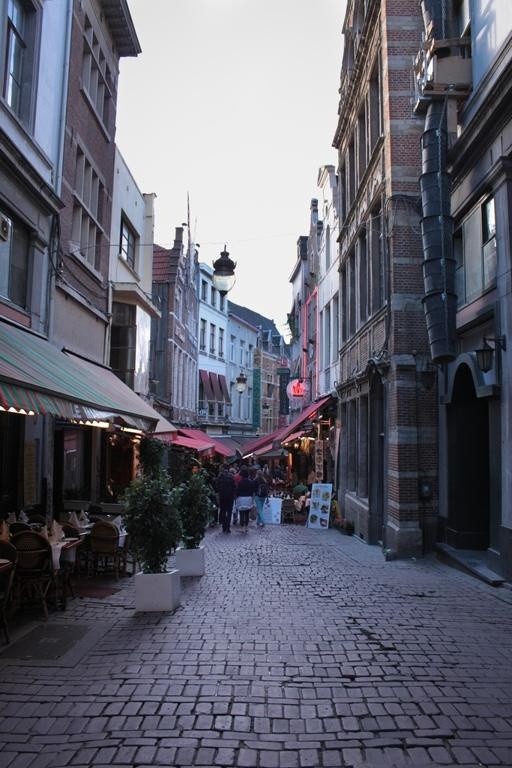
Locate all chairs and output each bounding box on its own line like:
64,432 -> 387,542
2,496 -> 135,643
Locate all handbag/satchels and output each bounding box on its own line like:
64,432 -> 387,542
235,495 -> 254,512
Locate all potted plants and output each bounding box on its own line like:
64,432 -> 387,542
122,438 -> 217,613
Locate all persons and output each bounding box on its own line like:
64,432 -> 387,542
192,456 -> 316,534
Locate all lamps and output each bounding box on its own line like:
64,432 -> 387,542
58,234 -> 236,297
475,335 -> 505,372
